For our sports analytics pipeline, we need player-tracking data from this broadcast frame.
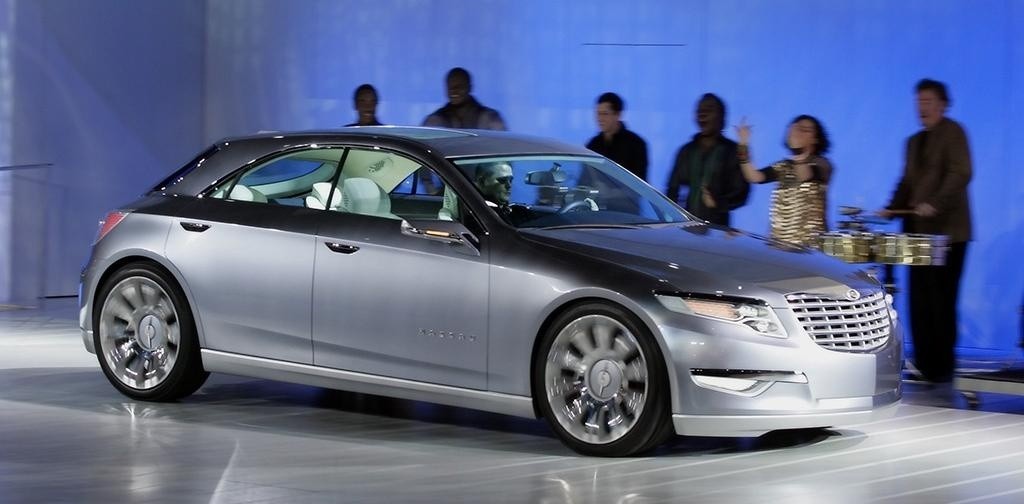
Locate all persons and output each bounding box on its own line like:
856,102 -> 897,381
871,76 -> 974,384
467,160 -> 599,231
666,91 -> 751,227
731,111 -> 832,251
342,83 -> 382,125
416,66 -> 506,195
572,90 -> 647,216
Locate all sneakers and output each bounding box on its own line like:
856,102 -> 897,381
900,359 -> 952,385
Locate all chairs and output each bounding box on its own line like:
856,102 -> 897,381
438,184 -> 459,222
224,185 -> 268,203
304,182 -> 341,213
344,176 -> 405,221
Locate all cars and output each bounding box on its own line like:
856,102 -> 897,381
77,124 -> 908,460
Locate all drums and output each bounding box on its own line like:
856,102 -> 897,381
872,232 -> 948,268
816,231 -> 872,265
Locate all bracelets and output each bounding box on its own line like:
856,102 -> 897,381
736,143 -> 750,165
